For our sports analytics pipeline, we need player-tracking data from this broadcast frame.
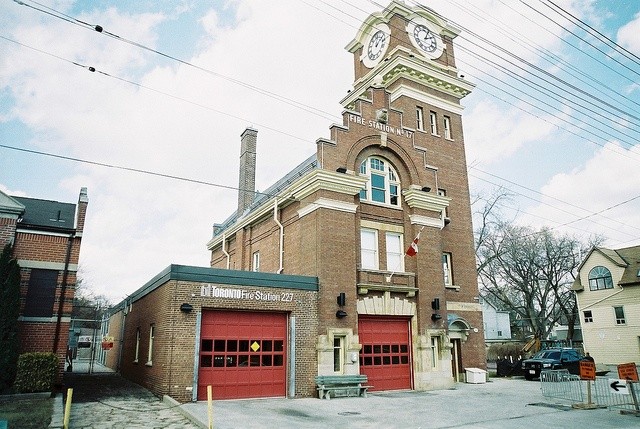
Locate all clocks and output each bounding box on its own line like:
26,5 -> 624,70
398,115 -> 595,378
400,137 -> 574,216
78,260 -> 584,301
367,30 -> 386,61
414,24 -> 437,52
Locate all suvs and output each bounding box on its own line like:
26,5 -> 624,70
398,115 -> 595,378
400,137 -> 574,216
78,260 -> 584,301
521,347 -> 584,380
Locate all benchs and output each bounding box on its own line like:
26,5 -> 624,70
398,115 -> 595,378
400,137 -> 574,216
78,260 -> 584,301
315,373 -> 374,400
